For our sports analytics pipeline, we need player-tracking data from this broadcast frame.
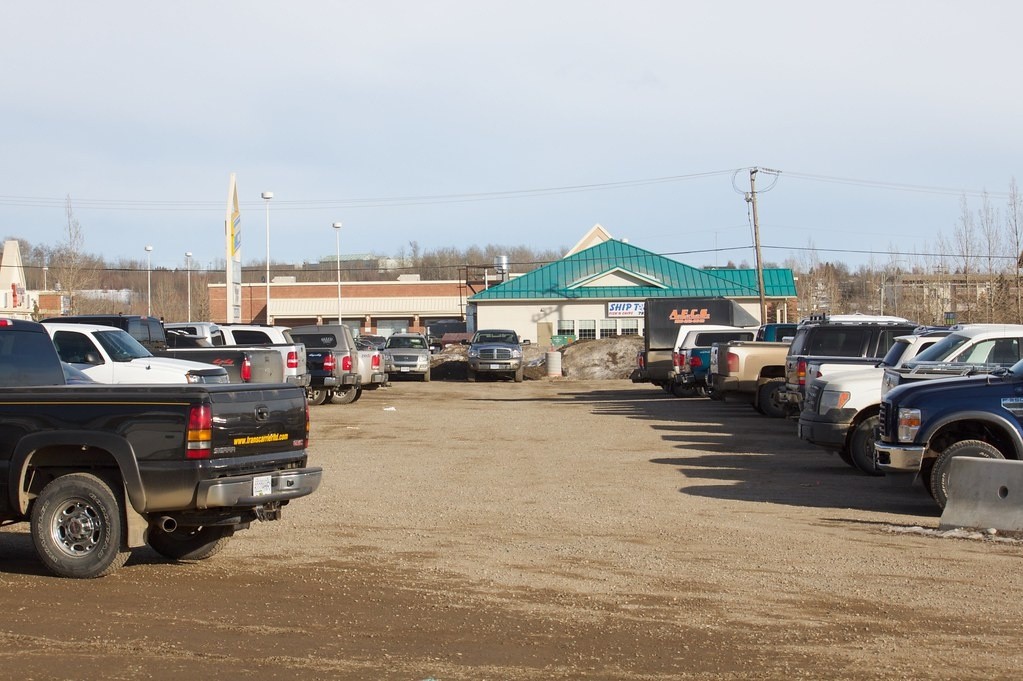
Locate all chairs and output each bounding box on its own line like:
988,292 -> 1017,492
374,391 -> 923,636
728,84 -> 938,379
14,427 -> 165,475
504,335 -> 515,343
480,336 -> 487,342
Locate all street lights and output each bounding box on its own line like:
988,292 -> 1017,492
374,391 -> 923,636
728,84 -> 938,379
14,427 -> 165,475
261,191 -> 273,326
42,266 -> 48,292
145,246 -> 152,317
186,252 -> 192,325
334,222 -> 341,326
881,259 -> 907,316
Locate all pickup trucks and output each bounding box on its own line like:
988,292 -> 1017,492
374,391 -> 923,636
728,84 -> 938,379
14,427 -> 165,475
690,315 -> 908,418
0,318 -> 322,578
37,316 -> 312,387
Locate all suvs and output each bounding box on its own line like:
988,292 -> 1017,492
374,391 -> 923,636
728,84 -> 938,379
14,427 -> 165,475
668,324 -> 758,397
461,329 -> 530,382
799,322 -> 1023,510
215,323 -> 435,405
779,313 -> 919,422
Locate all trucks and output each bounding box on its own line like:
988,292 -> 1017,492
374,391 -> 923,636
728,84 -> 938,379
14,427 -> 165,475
629,300 -> 760,390
